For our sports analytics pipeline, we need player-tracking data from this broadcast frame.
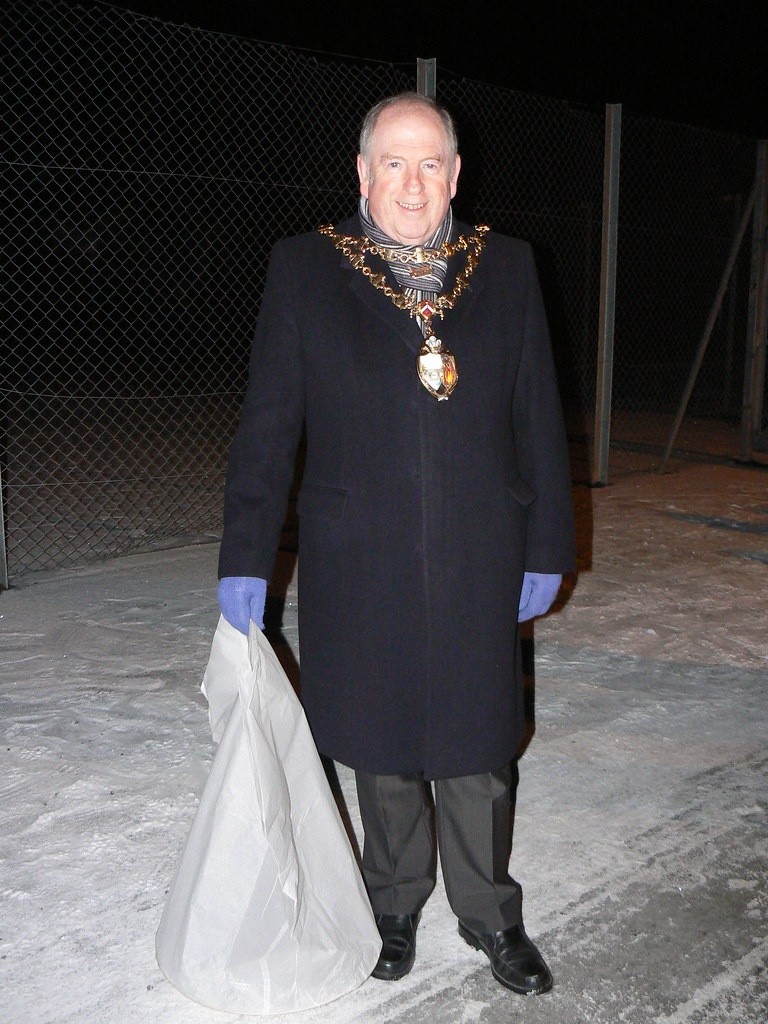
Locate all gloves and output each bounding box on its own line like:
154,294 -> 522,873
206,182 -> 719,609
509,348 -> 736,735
218,577 -> 267,633
518,573 -> 562,622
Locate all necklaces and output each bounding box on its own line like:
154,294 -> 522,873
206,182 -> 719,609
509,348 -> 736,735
314,220 -> 493,405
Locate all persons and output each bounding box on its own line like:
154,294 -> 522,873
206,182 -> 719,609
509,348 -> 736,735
212,87 -> 580,998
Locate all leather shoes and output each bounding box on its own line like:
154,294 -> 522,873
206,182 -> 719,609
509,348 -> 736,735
458,919 -> 553,996
370,909 -> 423,980
